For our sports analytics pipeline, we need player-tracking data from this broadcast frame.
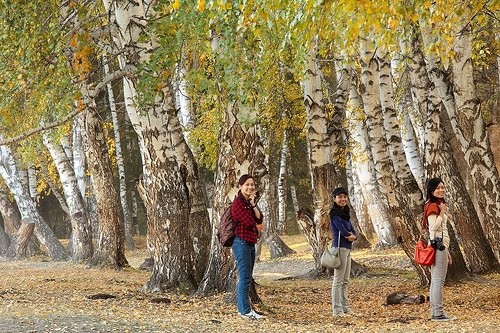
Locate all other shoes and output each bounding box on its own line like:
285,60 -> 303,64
345,312 -> 359,317
334,311 -> 350,317
431,315 -> 451,321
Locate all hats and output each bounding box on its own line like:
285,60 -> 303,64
332,187 -> 348,197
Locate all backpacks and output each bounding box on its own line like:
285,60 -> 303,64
218,197 -> 244,246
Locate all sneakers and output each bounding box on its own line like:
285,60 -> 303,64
238,309 -> 267,320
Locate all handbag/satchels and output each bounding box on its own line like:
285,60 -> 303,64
321,247 -> 341,268
415,240 -> 436,266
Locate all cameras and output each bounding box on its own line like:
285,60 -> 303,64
431,237 -> 445,251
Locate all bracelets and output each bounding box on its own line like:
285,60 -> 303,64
252,204 -> 256,208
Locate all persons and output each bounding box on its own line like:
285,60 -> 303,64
421,177 -> 453,321
330,187 -> 363,318
230,174 -> 264,318
